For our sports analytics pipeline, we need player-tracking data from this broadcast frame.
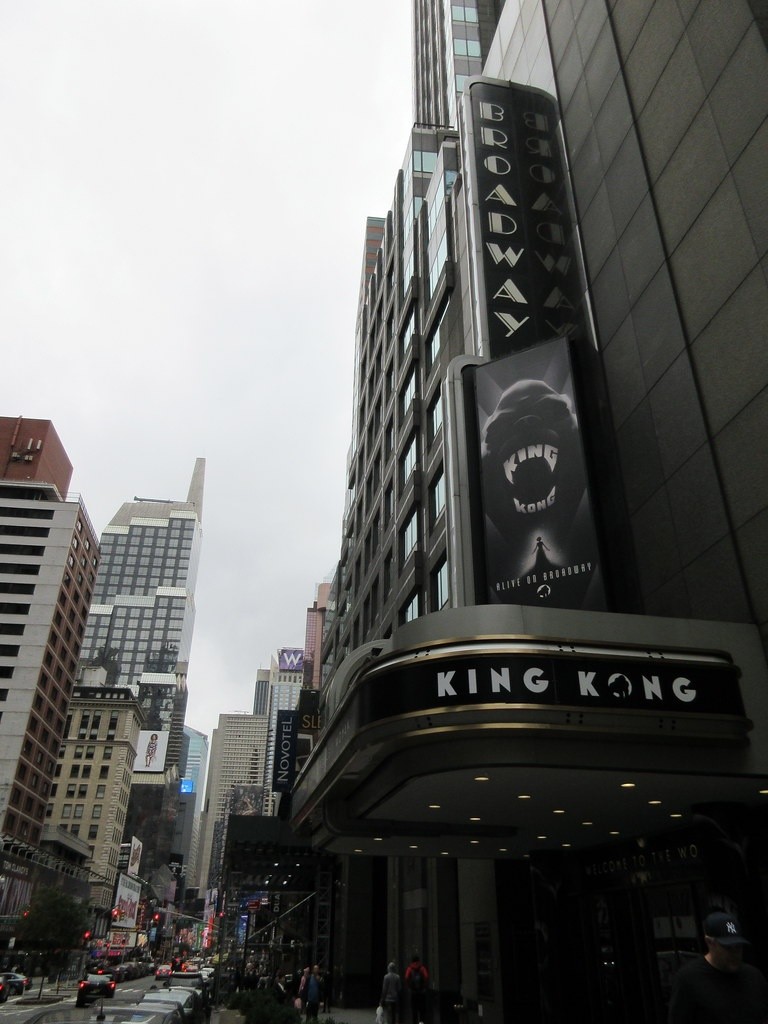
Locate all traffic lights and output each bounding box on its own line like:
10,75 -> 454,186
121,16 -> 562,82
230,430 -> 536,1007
151,911 -> 160,928
118,909 -> 126,922
111,908 -> 118,922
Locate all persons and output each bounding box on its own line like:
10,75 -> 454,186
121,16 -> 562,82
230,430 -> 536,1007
669,912 -> 768,1024
378,963 -> 401,1024
0,963 -> 23,973
145,734 -> 158,767
232,960 -> 333,1024
171,954 -> 186,972
405,957 -> 429,1024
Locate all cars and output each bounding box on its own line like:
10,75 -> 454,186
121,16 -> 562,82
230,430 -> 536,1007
21,1005 -> 181,1024
75,962 -> 218,1024
0,973 -> 33,1002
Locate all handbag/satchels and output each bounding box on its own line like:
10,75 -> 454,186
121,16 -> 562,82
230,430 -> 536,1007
294,996 -> 302,1009
376,1003 -> 385,1024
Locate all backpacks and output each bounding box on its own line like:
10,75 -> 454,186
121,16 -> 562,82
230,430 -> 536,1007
409,965 -> 424,990
